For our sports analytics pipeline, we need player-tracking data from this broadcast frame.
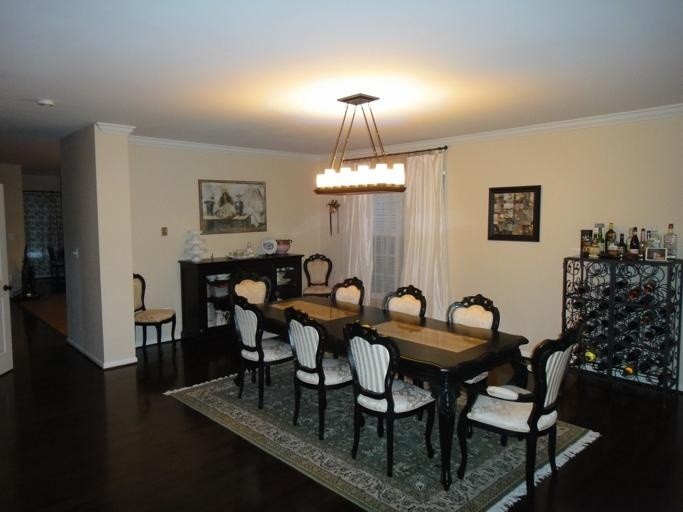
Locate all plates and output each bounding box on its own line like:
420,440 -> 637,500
225,252 -> 259,259
218,278 -> 231,280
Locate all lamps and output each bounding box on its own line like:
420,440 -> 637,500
311,91 -> 407,195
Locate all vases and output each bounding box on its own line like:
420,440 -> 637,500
277,240 -> 292,254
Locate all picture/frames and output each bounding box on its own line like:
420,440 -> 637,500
487,185 -> 541,242
645,247 -> 668,262
199,179 -> 267,235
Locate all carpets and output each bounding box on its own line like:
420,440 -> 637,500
164,354 -> 599,512
17,290 -> 66,335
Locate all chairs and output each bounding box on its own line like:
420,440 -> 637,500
284,307 -> 352,440
302,253 -> 332,297
230,272 -> 271,304
447,294 -> 500,439
230,295 -> 293,409
456,320 -> 587,495
132,273 -> 177,356
340,321 -> 435,476
383,285 -> 426,317
331,277 -> 364,306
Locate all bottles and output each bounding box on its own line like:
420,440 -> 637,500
606,223 -> 616,243
589,234 -> 601,259
646,232 -> 653,248
663,224 -> 677,258
618,233 -> 628,257
597,227 -> 606,255
606,234 -> 618,254
639,228 -> 646,256
626,228 -> 634,255
573,278 -> 683,380
630,227 -> 639,255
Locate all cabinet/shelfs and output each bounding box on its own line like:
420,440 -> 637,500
559,256 -> 682,456
177,254 -> 304,346
